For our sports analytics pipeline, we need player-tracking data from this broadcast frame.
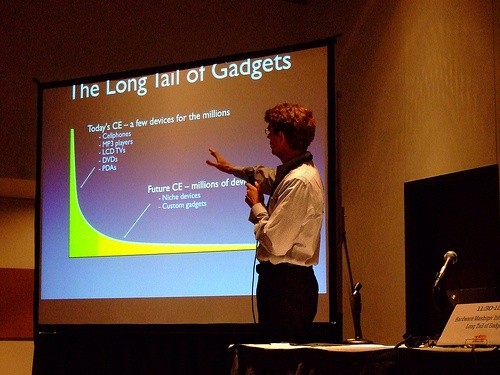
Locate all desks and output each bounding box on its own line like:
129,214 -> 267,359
234,344 -> 500,375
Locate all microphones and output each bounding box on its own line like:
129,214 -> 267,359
433,250 -> 459,292
244,166 -> 255,188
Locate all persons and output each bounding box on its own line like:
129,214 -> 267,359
206,100 -> 325,345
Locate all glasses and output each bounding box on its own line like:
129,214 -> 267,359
264,125 -> 280,136
